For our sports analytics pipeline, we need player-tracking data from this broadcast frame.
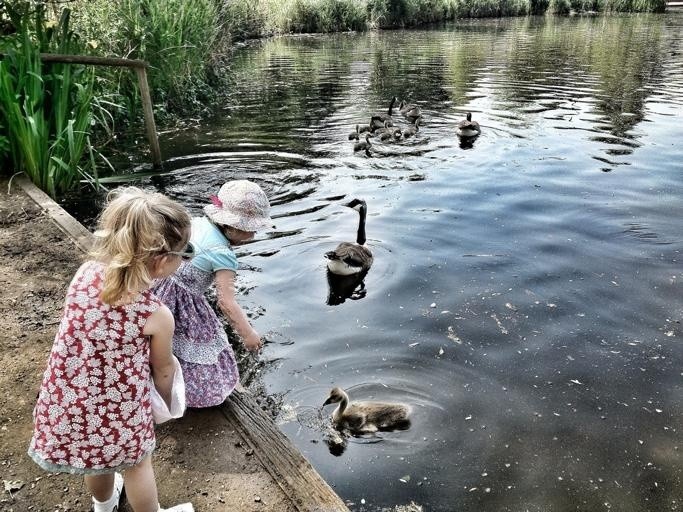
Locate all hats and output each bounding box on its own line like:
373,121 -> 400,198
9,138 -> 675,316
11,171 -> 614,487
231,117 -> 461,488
203,181 -> 273,233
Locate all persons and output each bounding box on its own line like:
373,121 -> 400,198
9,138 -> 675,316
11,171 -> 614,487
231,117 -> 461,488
151,179 -> 274,409
27,185 -> 196,512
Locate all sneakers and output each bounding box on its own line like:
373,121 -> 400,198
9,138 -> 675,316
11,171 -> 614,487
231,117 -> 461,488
92,471 -> 123,512
161,502 -> 197,512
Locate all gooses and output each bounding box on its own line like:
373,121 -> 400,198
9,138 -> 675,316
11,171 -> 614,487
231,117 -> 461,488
348,95 -> 423,151
321,386 -> 413,434
323,198 -> 374,276
455,112 -> 481,137
